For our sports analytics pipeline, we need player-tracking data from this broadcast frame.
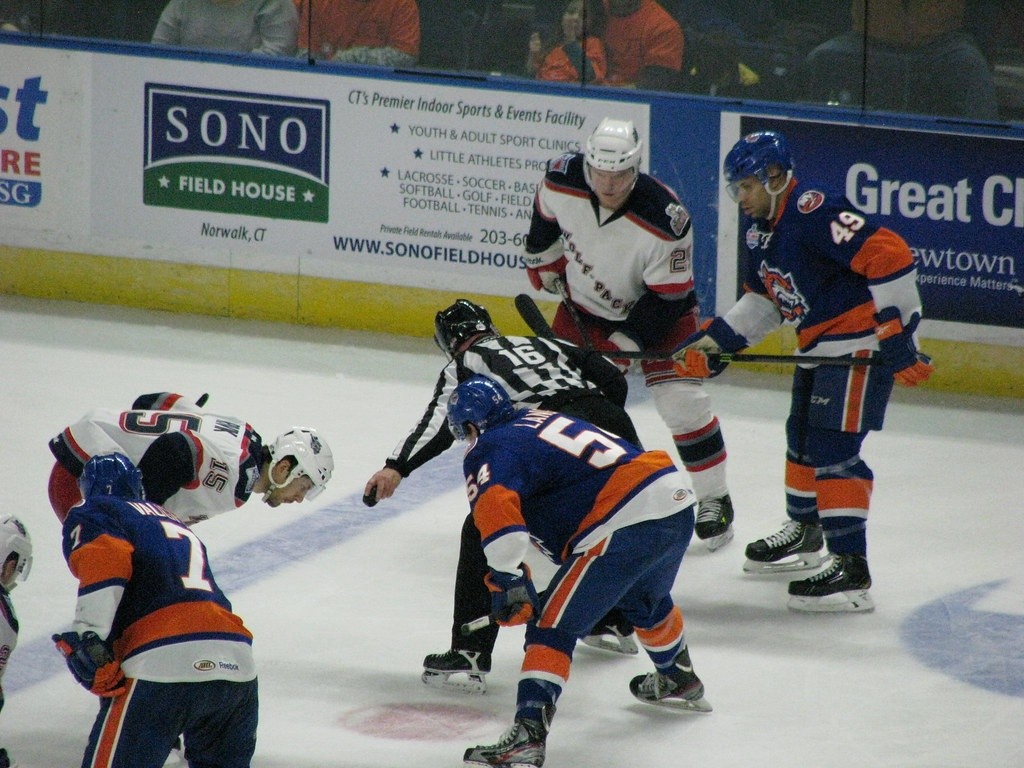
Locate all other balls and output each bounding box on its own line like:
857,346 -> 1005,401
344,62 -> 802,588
362,485 -> 379,507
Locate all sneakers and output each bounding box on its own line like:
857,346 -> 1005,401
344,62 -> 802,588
695,495 -> 736,553
463,720 -> 546,768
628,644 -> 714,712
422,649 -> 491,696
581,610 -> 639,655
786,554 -> 876,614
744,518 -> 824,574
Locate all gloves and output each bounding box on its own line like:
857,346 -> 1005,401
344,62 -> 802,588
872,306 -> 935,389
671,317 -> 748,379
595,331 -> 642,374
51,633 -> 127,698
524,238 -> 570,296
483,563 -> 540,625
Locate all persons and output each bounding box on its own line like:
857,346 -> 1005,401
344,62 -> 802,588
446,377 -> 713,767
48,389 -> 335,526
54,450 -> 259,768
670,131 -> 936,613
524,0 -> 682,92
288,0 -> 420,71
0,516 -> 32,767
363,299 -> 639,697
524,119 -> 735,553
152,0 -> 298,60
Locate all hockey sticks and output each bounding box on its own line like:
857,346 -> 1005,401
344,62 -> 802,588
554,279 -> 596,349
461,590 -> 547,636
512,293 -> 877,367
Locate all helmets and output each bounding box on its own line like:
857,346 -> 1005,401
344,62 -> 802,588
448,374 -> 515,441
261,426 -> 334,504
433,299 -> 500,363
77,453 -> 143,501
722,131 -> 794,184
583,117 -> 642,192
0,513 -> 33,591
